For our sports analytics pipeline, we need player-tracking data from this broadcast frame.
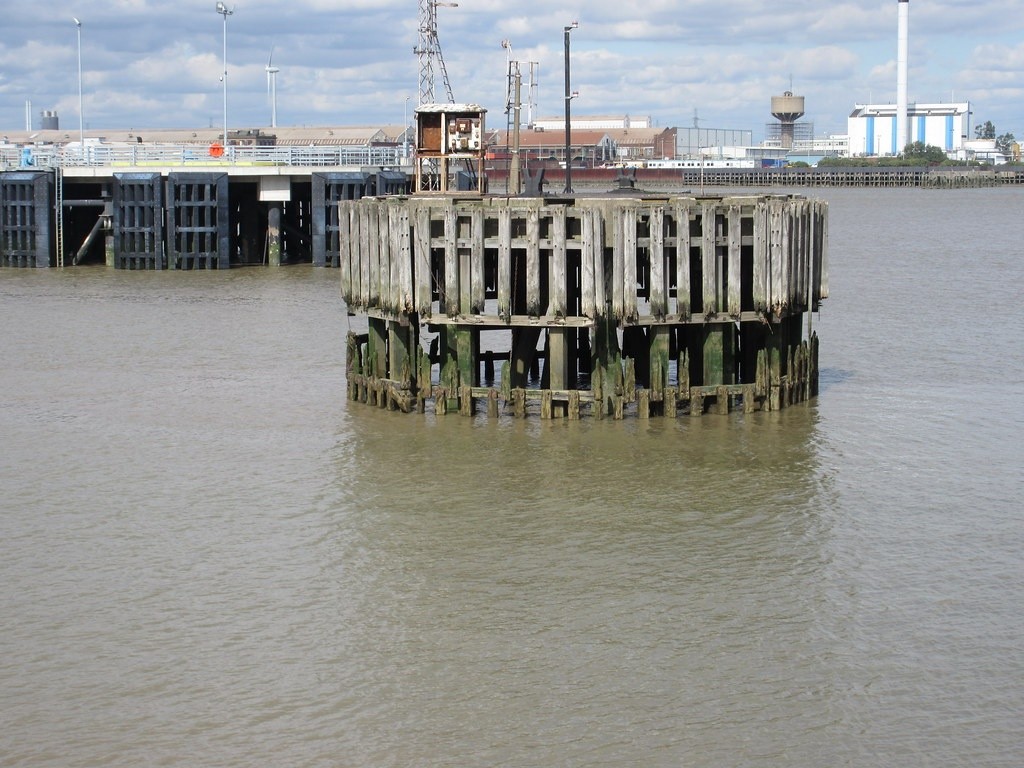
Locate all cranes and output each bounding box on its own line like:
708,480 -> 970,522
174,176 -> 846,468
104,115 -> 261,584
408,0 -> 456,108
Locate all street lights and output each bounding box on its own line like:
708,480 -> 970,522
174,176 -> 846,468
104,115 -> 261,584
403,96 -> 412,158
214,1 -> 233,157
73,17 -> 86,168
